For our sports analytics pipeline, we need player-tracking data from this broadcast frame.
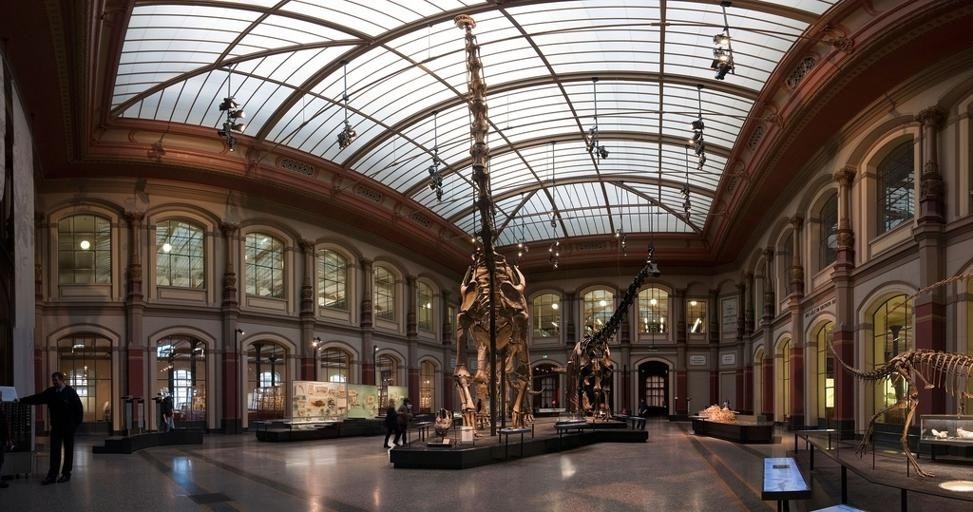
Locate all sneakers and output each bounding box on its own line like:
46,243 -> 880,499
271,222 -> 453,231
58,474 -> 71,482
40,475 -> 57,484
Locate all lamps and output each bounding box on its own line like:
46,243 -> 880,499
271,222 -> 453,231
335,61 -> 356,150
688,83 -> 710,170
234,326 -> 246,335
545,139 -> 562,270
312,336 -> 323,343
584,76 -> 610,164
678,171 -> 693,225
217,61 -> 246,153
428,109 -> 446,203
707,0 -> 737,81
614,179 -> 629,257
516,190 -> 528,260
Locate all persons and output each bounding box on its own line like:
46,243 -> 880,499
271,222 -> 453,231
393,398 -> 413,446
383,399 -> 397,449
14,372 -> 83,486
160,396 -> 175,433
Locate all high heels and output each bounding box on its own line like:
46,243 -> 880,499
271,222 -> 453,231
383,444 -> 391,449
